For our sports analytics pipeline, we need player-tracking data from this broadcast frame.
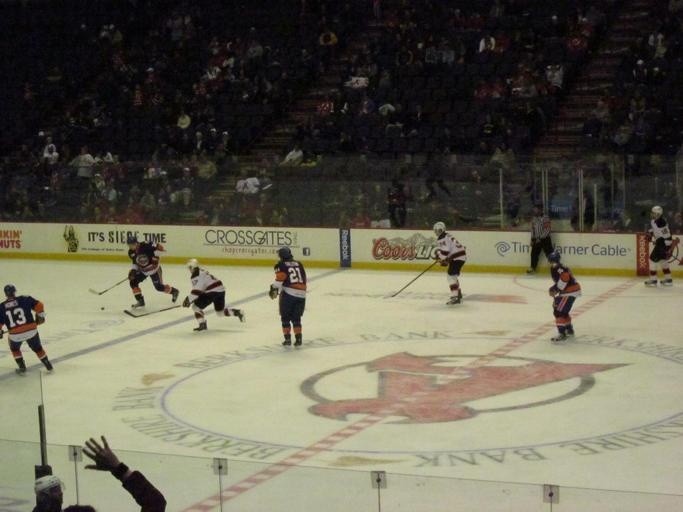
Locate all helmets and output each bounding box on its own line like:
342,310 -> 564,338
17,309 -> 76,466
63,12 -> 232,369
34,475 -> 61,494
187,258 -> 198,269
548,252 -> 559,263
433,221 -> 446,232
4,284 -> 15,294
651,206 -> 663,216
278,247 -> 293,259
126,237 -> 136,244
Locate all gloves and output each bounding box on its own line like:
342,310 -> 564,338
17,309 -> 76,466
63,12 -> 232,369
36,312 -> 45,325
550,285 -> 559,296
183,297 -> 191,307
269,284 -> 278,299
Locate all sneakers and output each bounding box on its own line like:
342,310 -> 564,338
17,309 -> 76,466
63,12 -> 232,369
447,299 -> 459,304
551,335 -> 565,341
527,268 -> 534,273
193,322 -> 206,331
170,287 -> 179,301
293,340 -> 301,346
644,280 -> 657,285
235,310 -> 243,322
133,303 -> 145,306
283,341 -> 291,345
661,279 -> 672,284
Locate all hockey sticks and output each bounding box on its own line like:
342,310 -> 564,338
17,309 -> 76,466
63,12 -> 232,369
384,260 -> 438,298
124,301 -> 195,318
89,277 -> 130,295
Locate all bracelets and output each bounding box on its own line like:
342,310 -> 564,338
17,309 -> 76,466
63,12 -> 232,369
112,462 -> 127,479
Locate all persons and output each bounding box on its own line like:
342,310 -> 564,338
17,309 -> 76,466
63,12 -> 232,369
266,247 -> 308,349
183,258 -> 245,333
31,462 -> 65,511
429,219 -> 466,303
645,201 -> 675,284
543,251 -> 581,342
1,0 -> 683,234
2,285 -> 53,374
62,435 -> 168,511
527,201 -> 560,273
122,231 -> 178,309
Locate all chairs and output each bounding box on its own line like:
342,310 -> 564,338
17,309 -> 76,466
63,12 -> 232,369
289,55 -> 510,177
584,34 -> 682,177
107,79 -> 276,167
